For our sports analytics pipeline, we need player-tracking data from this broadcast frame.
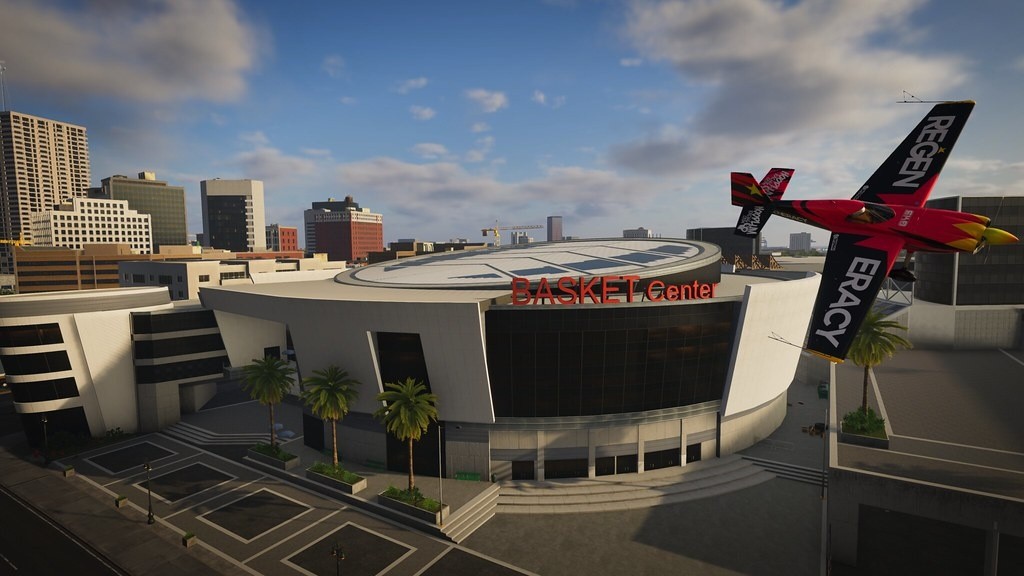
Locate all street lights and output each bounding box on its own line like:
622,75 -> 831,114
331,541 -> 345,576
41,415 -> 48,458
144,463 -> 155,524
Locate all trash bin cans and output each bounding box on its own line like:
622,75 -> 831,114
818,384 -> 828,400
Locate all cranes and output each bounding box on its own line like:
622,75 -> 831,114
481,220 -> 544,247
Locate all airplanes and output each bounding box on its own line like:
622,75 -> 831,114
730,89 -> 1020,362
